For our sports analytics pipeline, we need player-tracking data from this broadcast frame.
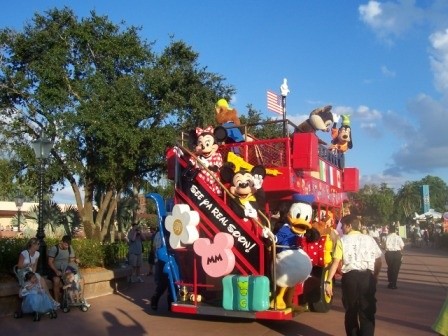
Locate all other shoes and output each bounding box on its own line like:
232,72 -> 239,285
71,300 -> 80,304
387,283 -> 398,289
145,271 -> 152,277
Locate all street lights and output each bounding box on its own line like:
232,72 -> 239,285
30,129 -> 54,275
12,194 -> 26,232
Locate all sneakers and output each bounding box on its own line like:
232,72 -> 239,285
168,305 -> 172,311
128,279 -> 135,283
85,301 -> 92,308
150,301 -> 159,311
54,302 -> 62,308
136,278 -> 146,283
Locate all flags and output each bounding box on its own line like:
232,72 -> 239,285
267,90 -> 283,114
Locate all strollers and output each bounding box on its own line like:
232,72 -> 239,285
12,264 -> 58,321
59,256 -> 89,314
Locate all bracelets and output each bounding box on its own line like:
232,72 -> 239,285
325,280 -> 331,284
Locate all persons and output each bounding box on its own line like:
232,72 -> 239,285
389,220 -> 423,250
148,225 -> 159,265
385,226 -> 405,289
325,215 -> 382,336
19,272 -> 54,313
361,225 -> 388,249
128,223 -> 146,283
49,235 -> 90,309
16,238 -> 60,311
62,272 -> 80,304
423,228 -> 430,248
148,231 -> 174,312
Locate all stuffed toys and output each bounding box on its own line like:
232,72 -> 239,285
190,126 -> 227,196
329,113 -> 353,169
215,99 -> 245,143
221,165 -> 265,221
312,210 -> 335,235
290,105 -> 333,145
262,193 -> 320,310
